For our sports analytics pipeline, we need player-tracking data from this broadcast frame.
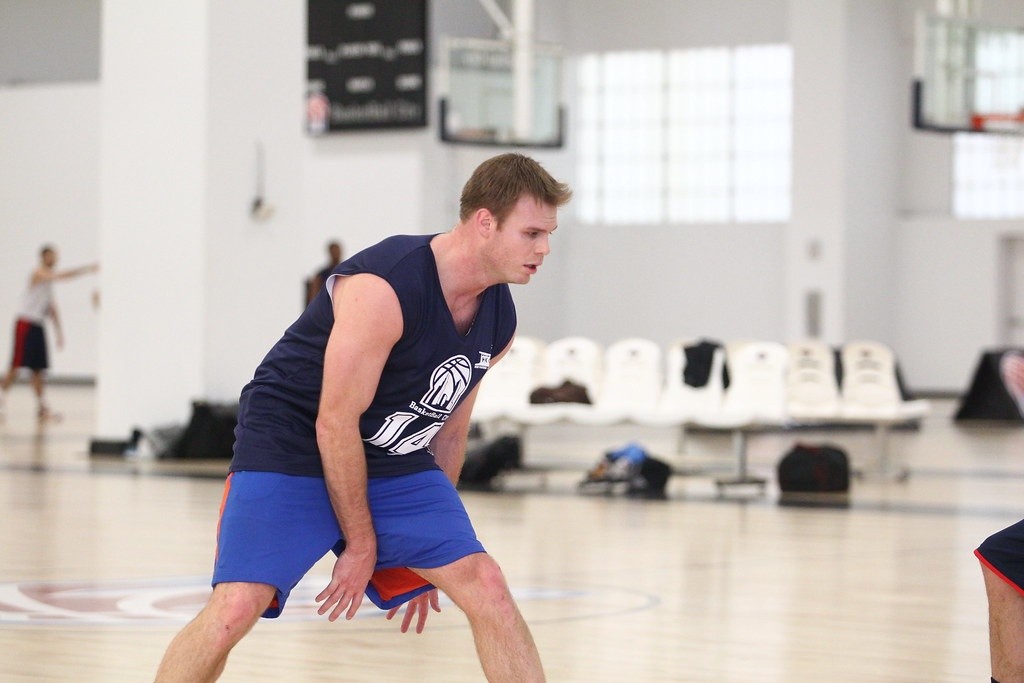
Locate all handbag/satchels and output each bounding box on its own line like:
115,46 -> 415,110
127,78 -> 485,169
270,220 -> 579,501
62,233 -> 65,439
779,445 -> 849,494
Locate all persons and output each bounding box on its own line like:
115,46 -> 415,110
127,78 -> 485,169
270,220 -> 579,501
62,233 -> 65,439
972,516 -> 1024,683
309,241 -> 344,302
1,243 -> 102,413
154,152 -> 570,680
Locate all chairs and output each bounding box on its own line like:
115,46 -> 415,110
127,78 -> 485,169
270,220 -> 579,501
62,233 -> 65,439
468,336 -> 933,494
